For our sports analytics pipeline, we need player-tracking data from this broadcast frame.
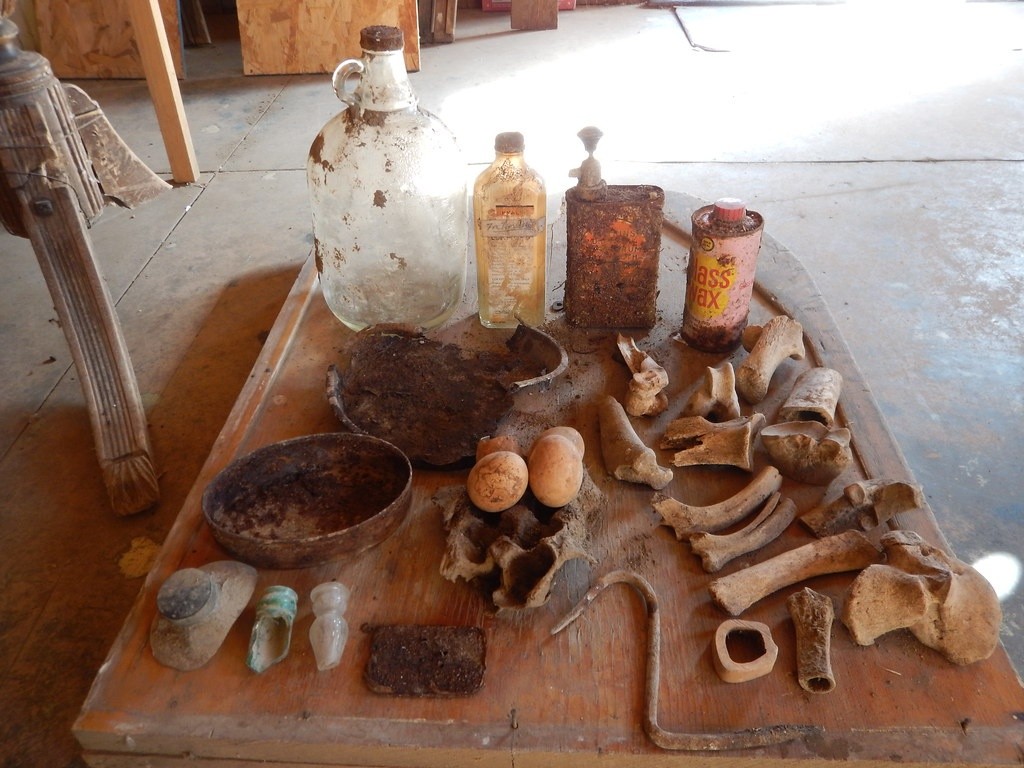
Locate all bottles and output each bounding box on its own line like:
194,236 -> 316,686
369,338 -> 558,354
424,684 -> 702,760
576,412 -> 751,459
305,25 -> 467,333
683,198 -> 764,351
473,132 -> 545,327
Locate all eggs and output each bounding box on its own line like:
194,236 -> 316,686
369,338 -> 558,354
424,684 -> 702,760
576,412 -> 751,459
467,426 -> 585,513
